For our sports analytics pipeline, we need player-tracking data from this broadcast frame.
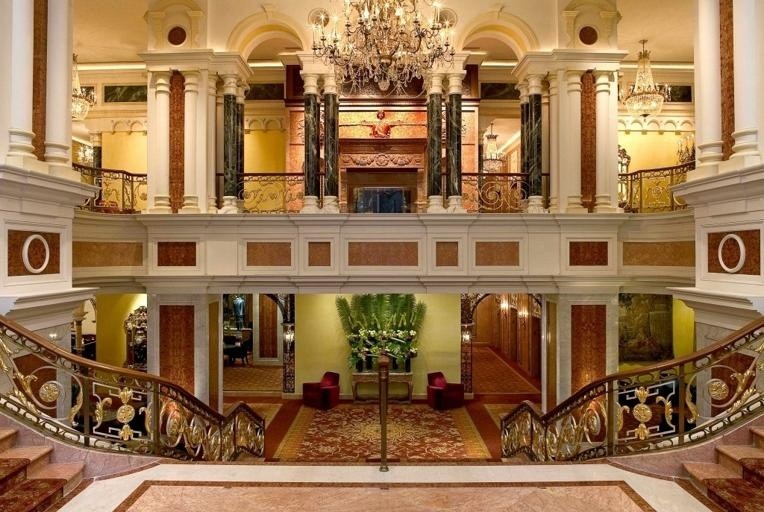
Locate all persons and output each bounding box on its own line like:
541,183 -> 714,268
360,112 -> 404,140
232,294 -> 246,331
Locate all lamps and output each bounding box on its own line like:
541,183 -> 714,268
309,1 -> 456,101
619,39 -> 673,124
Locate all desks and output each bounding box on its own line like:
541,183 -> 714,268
350,367 -> 414,406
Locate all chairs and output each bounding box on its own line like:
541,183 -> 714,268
303,371 -> 340,409
426,372 -> 464,411
224,335 -> 249,366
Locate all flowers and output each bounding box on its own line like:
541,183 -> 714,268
335,295 -> 427,366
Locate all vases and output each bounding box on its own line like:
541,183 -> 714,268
358,351 -> 410,373
